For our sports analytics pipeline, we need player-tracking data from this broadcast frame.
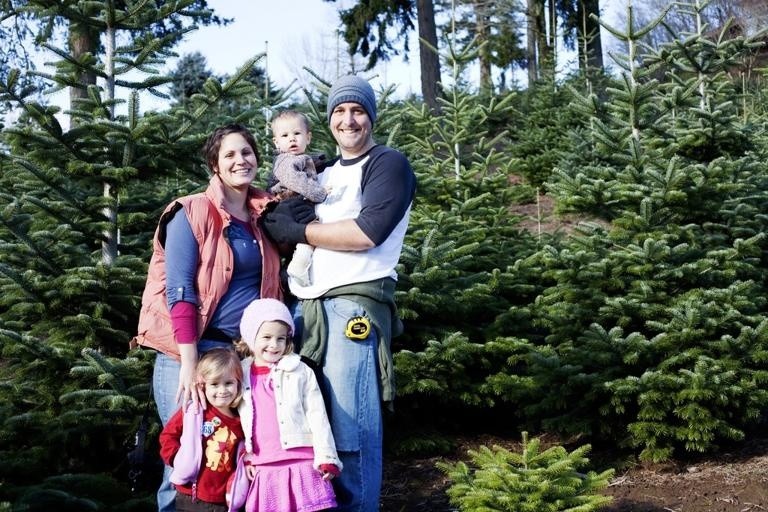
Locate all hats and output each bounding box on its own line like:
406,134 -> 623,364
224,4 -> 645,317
327,76 -> 376,129
240,298 -> 295,352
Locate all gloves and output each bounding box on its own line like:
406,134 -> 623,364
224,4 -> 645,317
263,199 -> 316,244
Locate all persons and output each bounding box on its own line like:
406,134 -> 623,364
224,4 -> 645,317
129,126 -> 285,512
237,297 -> 344,512
263,75 -> 418,512
266,109 -> 332,287
159,347 -> 243,512
